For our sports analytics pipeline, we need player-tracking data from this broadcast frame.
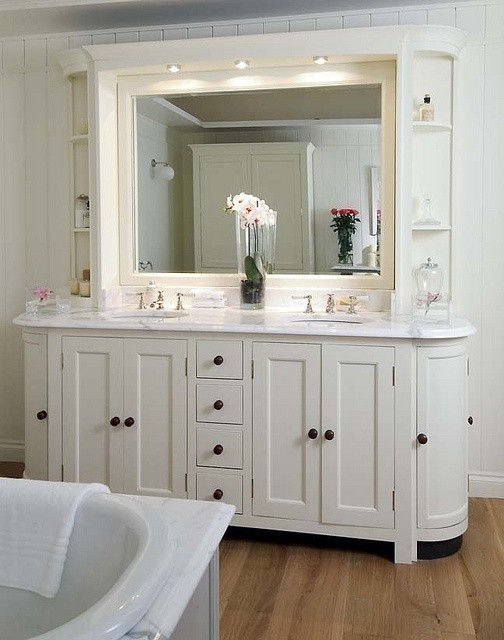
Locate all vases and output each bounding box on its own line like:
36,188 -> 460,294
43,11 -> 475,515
241,279 -> 263,305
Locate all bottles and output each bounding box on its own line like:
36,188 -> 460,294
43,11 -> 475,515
420,93 -> 434,122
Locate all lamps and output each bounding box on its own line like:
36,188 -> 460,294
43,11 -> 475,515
150,158 -> 177,182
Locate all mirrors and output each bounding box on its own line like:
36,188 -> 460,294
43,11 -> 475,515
117,59 -> 395,291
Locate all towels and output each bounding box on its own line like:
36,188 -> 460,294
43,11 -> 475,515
0,477 -> 109,599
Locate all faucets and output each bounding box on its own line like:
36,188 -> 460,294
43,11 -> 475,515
323,299 -> 335,313
150,296 -> 164,310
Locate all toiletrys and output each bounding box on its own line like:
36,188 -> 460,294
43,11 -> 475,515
419,92 -> 435,122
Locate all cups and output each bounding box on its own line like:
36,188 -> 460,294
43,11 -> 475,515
56,285 -> 71,315
25,286 -> 42,317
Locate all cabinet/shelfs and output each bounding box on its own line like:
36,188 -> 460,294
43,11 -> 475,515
56,24 -> 469,320
414,341 -> 473,541
186,142 -> 319,275
54,330 -> 188,498
15,305 -> 52,480
189,334 -> 250,517
251,340 -> 397,533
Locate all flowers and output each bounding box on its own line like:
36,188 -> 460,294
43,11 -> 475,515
329,206 -> 361,266
223,191 -> 268,273
253,208 -> 277,253
